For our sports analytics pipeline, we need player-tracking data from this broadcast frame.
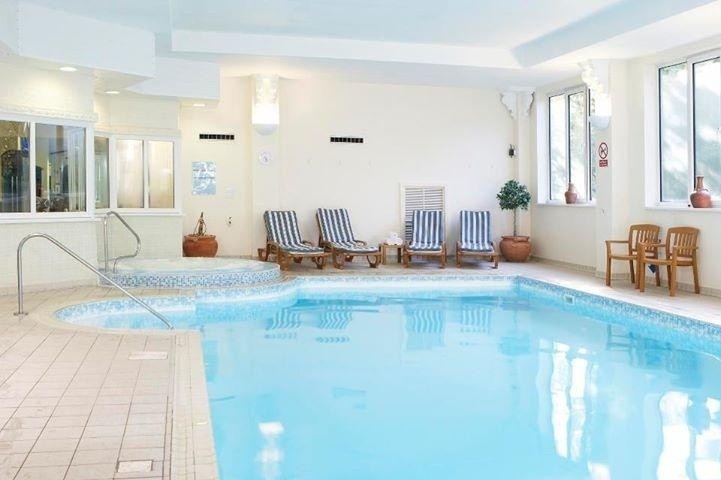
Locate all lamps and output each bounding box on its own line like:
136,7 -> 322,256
508,145 -> 516,157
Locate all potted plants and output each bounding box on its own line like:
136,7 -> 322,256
496,182 -> 532,263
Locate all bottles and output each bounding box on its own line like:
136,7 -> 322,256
565,182 -> 577,204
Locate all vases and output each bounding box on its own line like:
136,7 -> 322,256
564,183 -> 578,203
183,234 -> 219,257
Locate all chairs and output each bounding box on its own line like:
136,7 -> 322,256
606,224 -> 701,296
257,208 -> 499,270
